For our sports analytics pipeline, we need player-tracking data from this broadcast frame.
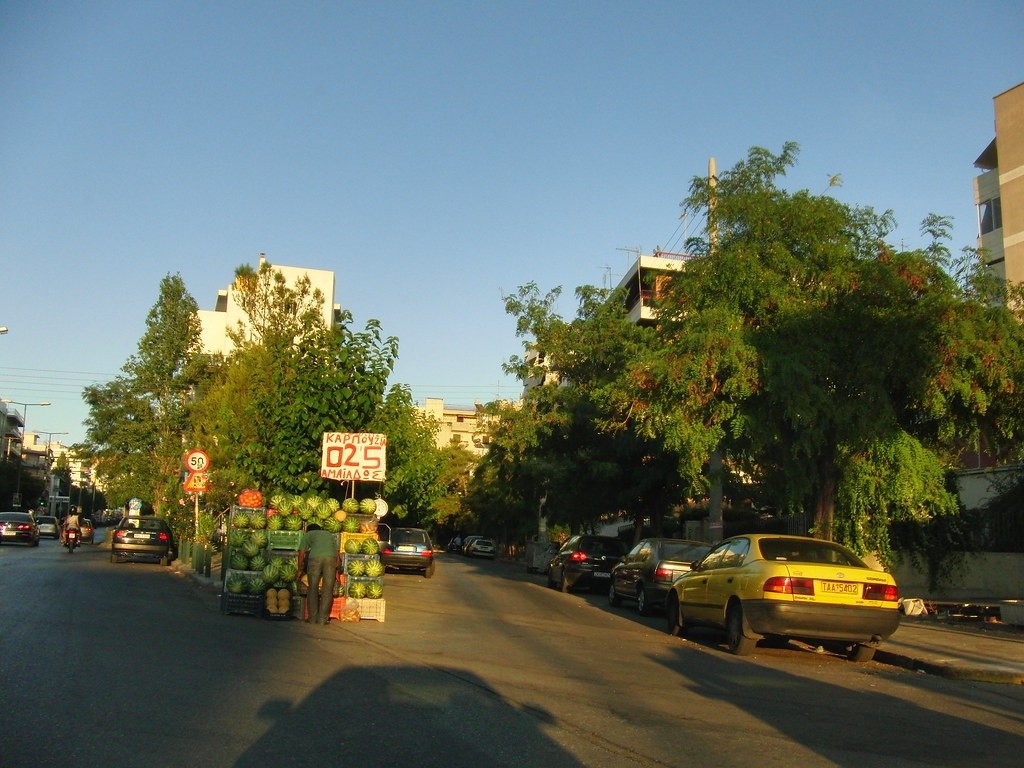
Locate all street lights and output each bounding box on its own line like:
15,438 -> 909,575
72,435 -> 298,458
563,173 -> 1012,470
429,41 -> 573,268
1,399 -> 51,511
31,429 -> 69,515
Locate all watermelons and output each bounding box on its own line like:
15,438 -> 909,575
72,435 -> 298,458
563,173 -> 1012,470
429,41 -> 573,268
225,495 -> 385,599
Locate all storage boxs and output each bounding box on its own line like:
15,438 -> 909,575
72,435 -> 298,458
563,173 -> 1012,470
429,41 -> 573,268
220,504 -> 386,622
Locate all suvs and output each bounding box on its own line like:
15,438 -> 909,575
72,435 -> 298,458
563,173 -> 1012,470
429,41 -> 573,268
546,534 -> 627,594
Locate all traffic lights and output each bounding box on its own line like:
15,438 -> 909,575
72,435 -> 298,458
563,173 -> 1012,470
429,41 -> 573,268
39,499 -> 48,507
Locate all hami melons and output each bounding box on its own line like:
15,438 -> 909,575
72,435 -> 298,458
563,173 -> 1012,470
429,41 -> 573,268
267,588 -> 291,614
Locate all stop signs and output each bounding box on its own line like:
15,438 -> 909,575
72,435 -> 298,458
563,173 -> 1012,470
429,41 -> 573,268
185,450 -> 210,474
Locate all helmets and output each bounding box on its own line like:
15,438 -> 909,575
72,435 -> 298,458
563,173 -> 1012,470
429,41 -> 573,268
70,506 -> 77,512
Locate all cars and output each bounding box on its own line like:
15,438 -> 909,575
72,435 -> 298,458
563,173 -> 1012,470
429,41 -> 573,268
0,511 -> 40,547
447,535 -> 497,560
109,514 -> 172,566
662,534 -> 902,661
79,517 -> 95,545
33,516 -> 60,541
607,537 -> 714,616
376,527 -> 436,579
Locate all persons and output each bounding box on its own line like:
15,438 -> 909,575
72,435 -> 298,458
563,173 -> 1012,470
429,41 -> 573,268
296,523 -> 342,625
454,534 -> 462,551
63,505 -> 82,547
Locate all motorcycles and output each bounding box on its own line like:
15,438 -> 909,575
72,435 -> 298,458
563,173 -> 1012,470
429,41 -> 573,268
64,527 -> 79,553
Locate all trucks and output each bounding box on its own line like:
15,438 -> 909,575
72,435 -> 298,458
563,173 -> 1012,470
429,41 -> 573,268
525,541 -> 561,573
